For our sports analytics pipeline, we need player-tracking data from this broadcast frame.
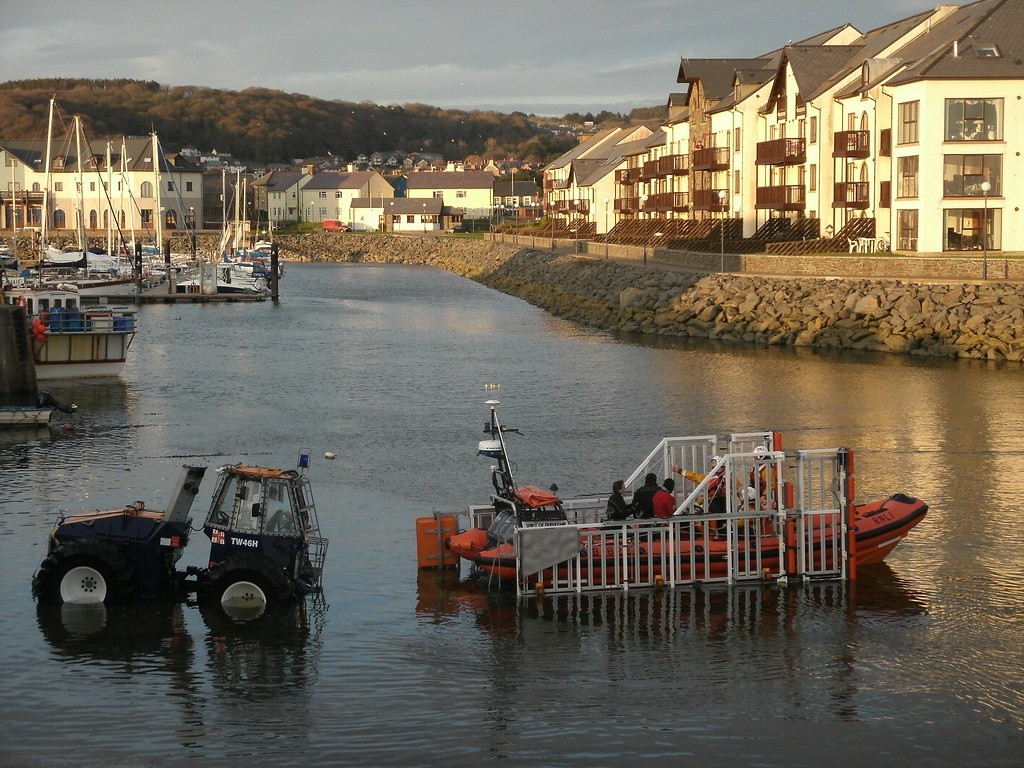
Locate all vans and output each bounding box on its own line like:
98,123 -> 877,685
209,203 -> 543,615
322,220 -> 352,233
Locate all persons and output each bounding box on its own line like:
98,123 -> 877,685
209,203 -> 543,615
606,446 -> 777,534
234,260 -> 237,263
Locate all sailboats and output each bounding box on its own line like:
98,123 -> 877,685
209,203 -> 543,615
0,92 -> 285,294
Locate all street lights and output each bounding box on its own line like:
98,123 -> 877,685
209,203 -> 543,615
514,203 -> 520,245
422,202 -> 426,233
488,205 -> 505,245
718,190 -> 726,272
471,205 -> 475,233
550,200 -> 556,250
980,181 -> 992,282
573,200 -> 580,254
640,194 -> 648,265
604,196 -> 610,260
530,201 -> 535,247
390,201 -> 394,234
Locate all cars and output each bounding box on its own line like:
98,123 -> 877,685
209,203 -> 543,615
273,225 -> 282,231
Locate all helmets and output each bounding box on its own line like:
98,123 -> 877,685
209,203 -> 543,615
711,455 -> 726,467
754,446 -> 768,459
741,487 -> 756,501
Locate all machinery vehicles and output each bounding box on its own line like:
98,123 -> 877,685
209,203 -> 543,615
37,445 -> 330,629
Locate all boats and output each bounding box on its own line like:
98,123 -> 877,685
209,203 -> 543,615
441,384 -> 930,587
0,264 -> 139,381
